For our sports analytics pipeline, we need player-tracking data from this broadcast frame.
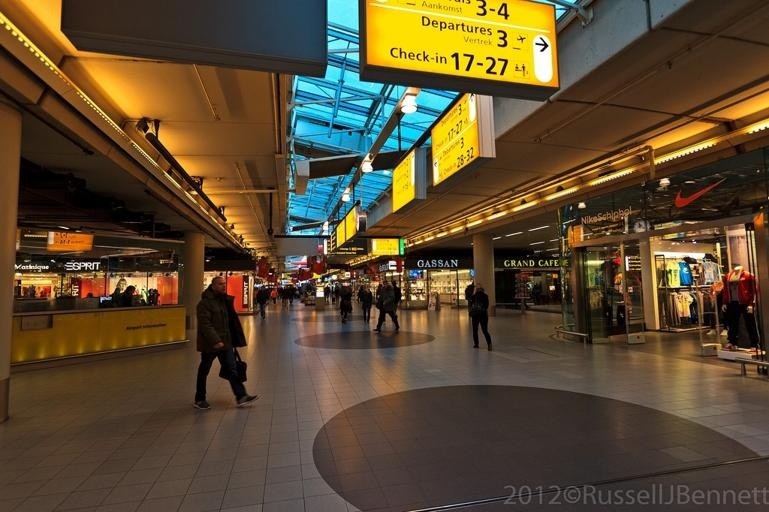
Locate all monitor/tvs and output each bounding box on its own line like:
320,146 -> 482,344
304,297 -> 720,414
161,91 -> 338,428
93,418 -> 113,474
408,270 -> 423,279
99,296 -> 112,304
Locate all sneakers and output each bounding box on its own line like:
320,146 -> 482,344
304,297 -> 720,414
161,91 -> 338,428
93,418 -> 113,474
236,392 -> 263,409
473,344 -> 479,348
193,400 -> 212,410
488,343 -> 492,351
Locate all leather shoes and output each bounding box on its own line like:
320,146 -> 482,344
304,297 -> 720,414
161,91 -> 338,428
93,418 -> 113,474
373,327 -> 382,332
395,326 -> 399,330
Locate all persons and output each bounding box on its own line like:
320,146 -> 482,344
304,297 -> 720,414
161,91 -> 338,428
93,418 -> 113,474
253,280 -> 342,307
391,280 -> 402,320
190,275 -> 264,410
256,286 -> 270,321
112,278 -> 127,304
469,284 -> 493,351
119,285 -> 136,306
361,289 -> 372,324
468,282 -> 475,316
375,283 -> 384,303
339,287 -> 352,324
357,285 -> 365,303
373,279 -> 400,332
720,265 -> 762,352
705,278 -> 731,336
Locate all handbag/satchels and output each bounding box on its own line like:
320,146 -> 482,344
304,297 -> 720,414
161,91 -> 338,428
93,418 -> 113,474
219,357 -> 248,383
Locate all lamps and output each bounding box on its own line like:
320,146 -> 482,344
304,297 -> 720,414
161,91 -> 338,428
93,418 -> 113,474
322,222 -> 329,231
360,159 -> 374,174
342,193 -> 351,202
398,93 -> 418,114
